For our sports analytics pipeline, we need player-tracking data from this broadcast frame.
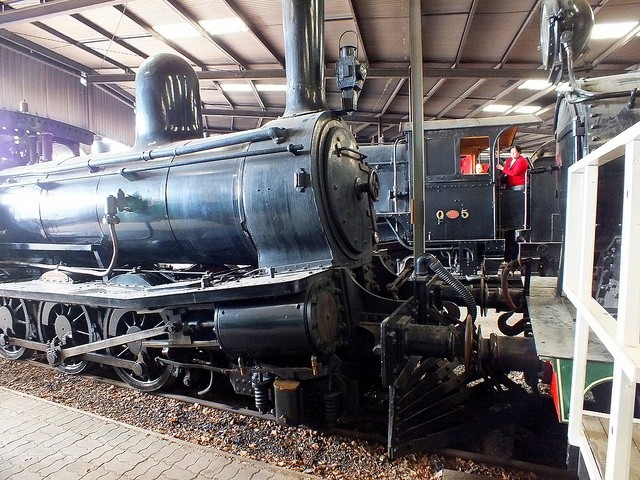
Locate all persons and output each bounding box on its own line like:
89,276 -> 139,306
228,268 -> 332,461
486,149 -> 505,175
496,145 -> 529,191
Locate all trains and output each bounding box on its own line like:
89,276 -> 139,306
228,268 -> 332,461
0,0 -> 640,461
357,114 -> 559,277
